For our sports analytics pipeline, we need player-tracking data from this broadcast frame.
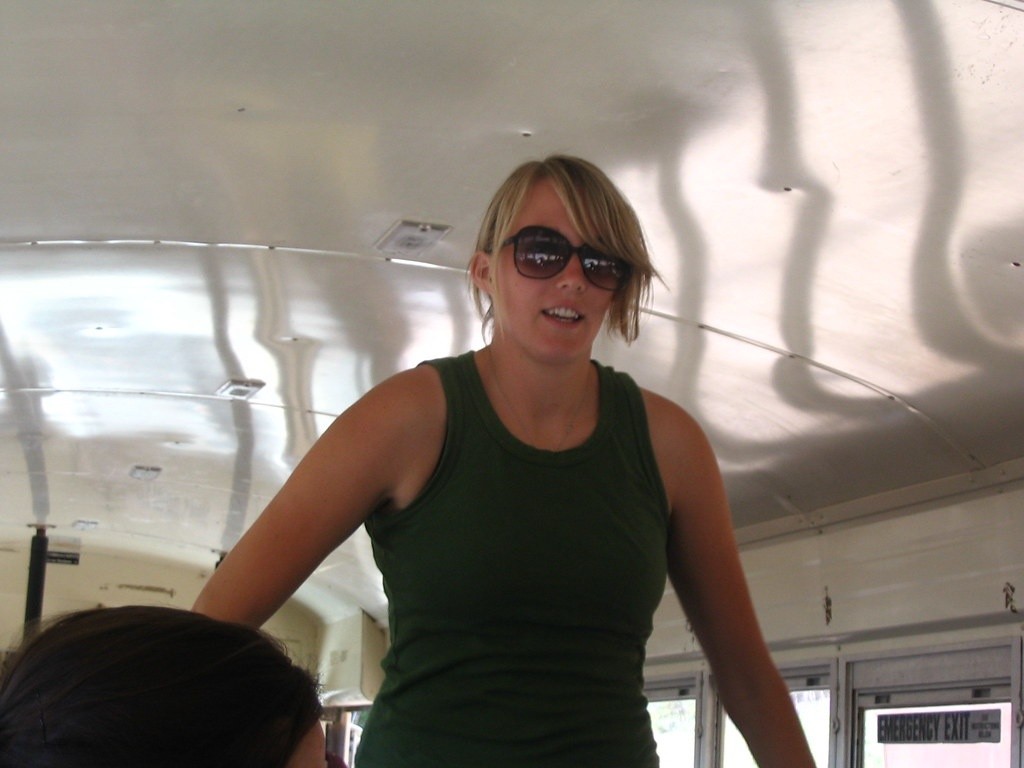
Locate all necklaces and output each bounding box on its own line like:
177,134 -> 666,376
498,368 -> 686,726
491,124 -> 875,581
488,346 -> 590,451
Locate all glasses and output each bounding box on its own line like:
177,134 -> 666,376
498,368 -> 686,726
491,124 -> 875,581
484,224 -> 635,291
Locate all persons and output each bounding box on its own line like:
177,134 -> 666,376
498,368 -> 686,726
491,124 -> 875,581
0,607 -> 326,767
194,156 -> 818,767
